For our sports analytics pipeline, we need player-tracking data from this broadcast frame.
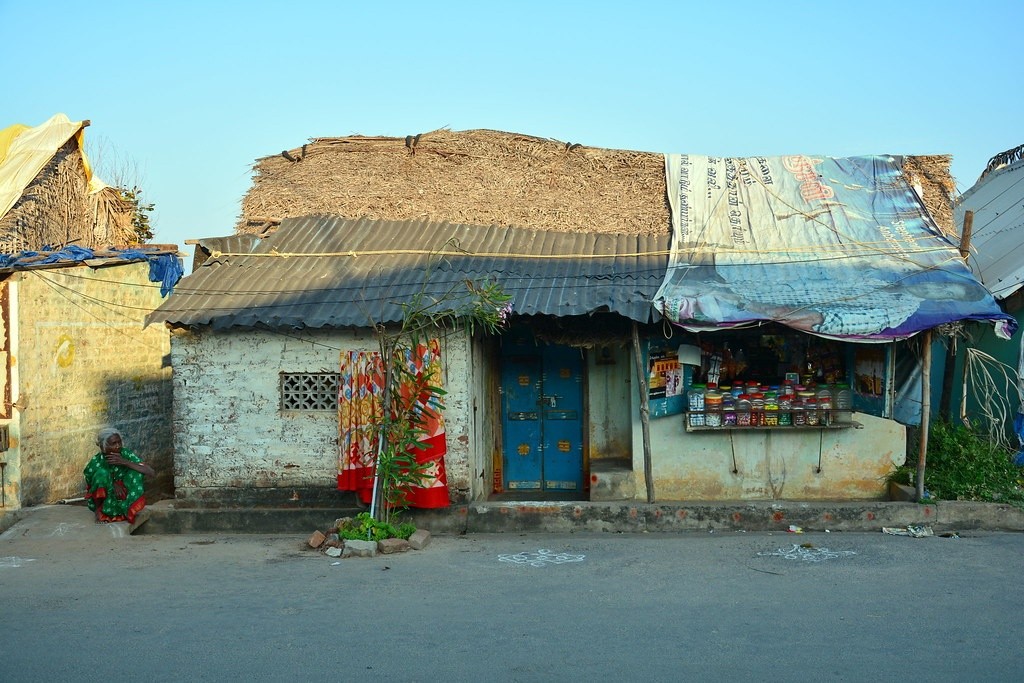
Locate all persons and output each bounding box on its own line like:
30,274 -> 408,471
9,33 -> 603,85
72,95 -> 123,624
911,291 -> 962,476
83,428 -> 156,524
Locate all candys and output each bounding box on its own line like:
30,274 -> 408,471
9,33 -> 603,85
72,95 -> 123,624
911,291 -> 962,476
736,411 -> 819,426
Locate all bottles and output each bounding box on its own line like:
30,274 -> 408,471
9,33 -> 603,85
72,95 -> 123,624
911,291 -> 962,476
686,375 -> 852,428
